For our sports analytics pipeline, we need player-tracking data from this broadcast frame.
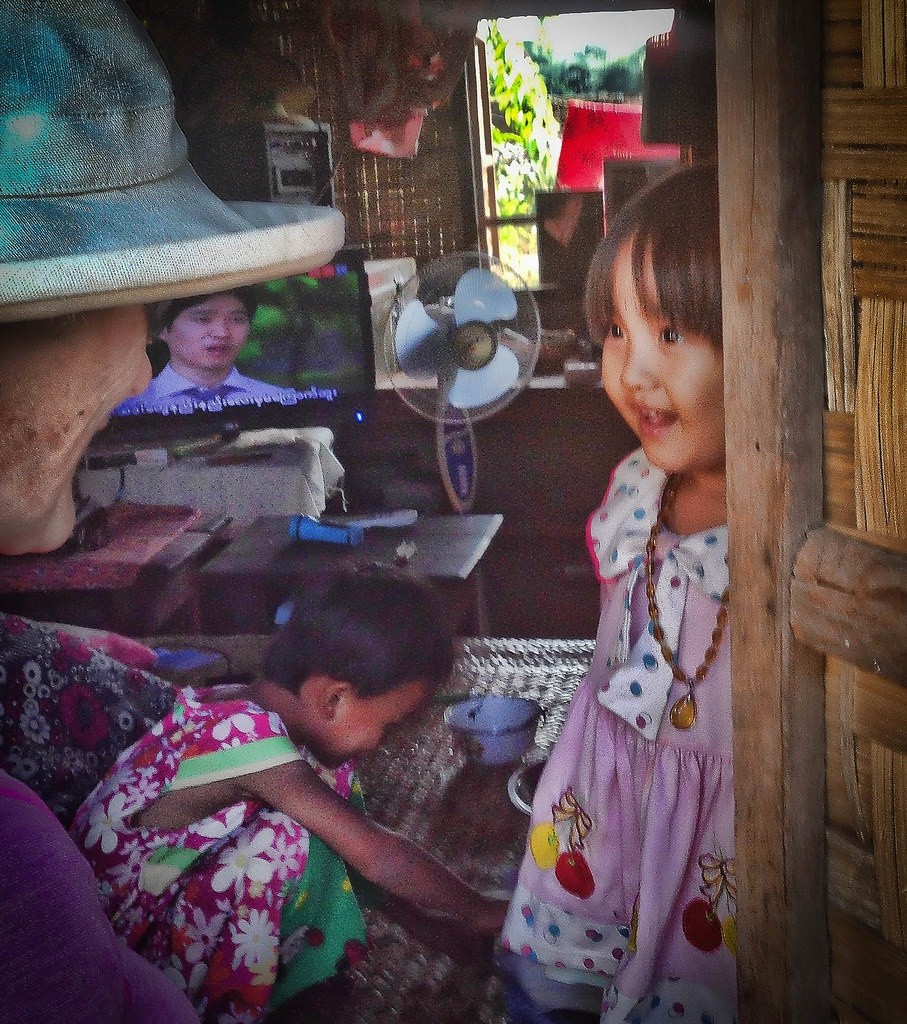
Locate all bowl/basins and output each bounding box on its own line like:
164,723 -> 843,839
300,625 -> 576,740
443,697 -> 541,765
564,363 -> 602,387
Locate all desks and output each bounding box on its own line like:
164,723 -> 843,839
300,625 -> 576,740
0,504 -> 233,642
198,511 -> 505,640
74,425 -> 346,520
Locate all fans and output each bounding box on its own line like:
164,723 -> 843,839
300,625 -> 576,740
383,251 -> 545,638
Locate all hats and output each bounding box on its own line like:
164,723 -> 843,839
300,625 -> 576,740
0,0 -> 344,321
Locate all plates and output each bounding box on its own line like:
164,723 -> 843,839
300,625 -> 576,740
507,760 -> 546,815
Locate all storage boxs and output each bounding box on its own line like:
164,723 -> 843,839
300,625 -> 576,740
602,156 -> 680,233
536,189 -> 604,285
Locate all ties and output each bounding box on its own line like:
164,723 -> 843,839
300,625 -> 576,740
188,386 -> 231,411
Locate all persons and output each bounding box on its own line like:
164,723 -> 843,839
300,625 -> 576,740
115,288 -> 300,423
498,161 -> 737,1024
3,0 -> 520,1024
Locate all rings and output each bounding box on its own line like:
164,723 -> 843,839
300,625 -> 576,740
645,472 -> 730,729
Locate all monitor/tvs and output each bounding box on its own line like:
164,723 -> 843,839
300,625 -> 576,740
110,253 -> 375,424
